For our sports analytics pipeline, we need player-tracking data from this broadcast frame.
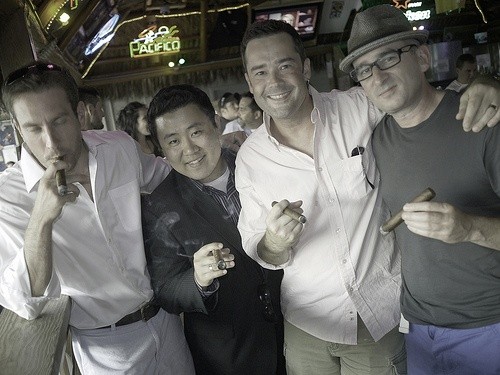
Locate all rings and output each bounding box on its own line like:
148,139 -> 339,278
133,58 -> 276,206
489,104 -> 497,110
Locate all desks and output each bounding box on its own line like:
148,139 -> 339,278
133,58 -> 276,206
0,295 -> 72,375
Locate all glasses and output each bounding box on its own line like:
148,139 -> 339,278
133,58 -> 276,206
348,44 -> 419,83
2,62 -> 63,114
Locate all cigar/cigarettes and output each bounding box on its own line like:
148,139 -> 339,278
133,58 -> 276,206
53,156 -> 69,198
379,186 -> 436,239
271,200 -> 307,224
211,248 -> 227,271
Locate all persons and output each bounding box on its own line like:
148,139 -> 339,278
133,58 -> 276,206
0,161 -> 15,174
76,86 -> 107,131
218,90 -> 264,156
443,52 -> 479,95
235,19 -> 499,375
213,93 -> 241,133
339,3 -> 500,375
0,60 -> 248,374
137,82 -> 288,375
114,99 -> 166,159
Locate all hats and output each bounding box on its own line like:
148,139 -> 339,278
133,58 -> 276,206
339,4 -> 429,74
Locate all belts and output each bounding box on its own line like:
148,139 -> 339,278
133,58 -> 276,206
97,295 -> 161,330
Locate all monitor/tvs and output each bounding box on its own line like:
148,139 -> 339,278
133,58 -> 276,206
425,40 -> 463,83
